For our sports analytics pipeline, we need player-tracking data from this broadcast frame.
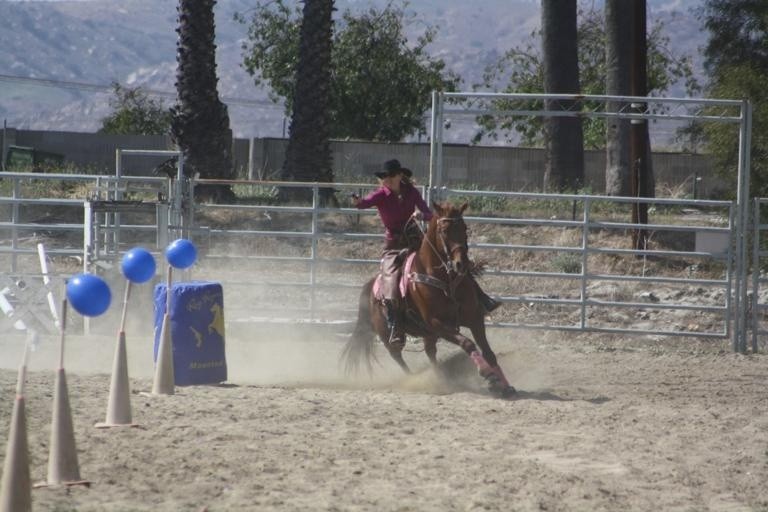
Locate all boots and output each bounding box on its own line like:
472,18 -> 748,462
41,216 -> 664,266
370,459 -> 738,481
383,299 -> 404,347
474,281 -> 501,312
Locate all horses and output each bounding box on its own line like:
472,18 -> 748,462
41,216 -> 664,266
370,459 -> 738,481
338,199 -> 516,399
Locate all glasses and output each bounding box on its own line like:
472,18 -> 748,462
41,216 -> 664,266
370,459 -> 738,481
382,173 -> 396,178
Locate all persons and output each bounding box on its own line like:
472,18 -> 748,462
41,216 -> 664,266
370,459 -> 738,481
351,159 -> 434,345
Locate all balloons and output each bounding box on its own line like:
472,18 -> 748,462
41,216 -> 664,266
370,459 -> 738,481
166,240 -> 197,269
66,273 -> 111,317
121,247 -> 157,283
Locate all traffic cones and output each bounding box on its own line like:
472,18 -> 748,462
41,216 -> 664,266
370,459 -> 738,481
32,365 -> 98,488
92,329 -> 140,429
139,311 -> 183,397
0,393 -> 36,512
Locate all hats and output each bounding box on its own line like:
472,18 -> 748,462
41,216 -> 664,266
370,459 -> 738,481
373,158 -> 413,178
